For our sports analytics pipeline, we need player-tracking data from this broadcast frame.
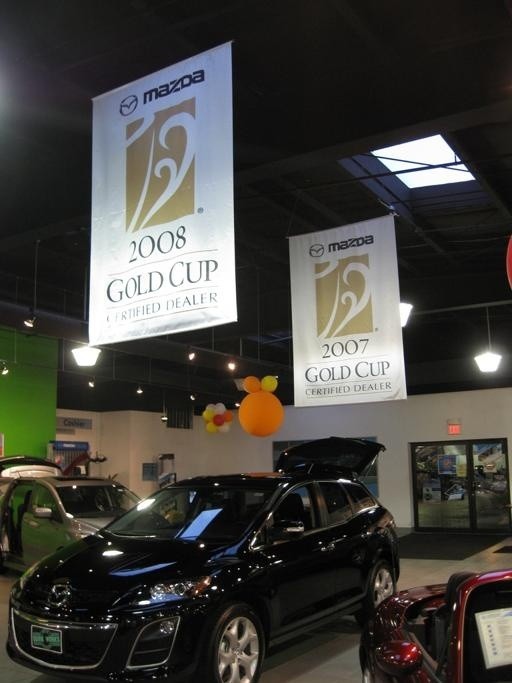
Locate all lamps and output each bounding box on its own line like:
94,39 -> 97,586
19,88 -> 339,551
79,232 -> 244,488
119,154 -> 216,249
22,240 -> 39,330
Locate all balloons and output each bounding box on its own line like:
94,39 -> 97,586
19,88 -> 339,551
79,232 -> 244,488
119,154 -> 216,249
202,402 -> 234,434
238,376 -> 284,437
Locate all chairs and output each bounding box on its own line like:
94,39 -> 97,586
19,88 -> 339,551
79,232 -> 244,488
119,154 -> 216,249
270,492 -> 305,526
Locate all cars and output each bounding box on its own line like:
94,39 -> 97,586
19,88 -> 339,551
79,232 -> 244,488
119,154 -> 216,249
360,570 -> 512,683
6,436 -> 406,682
0,456 -> 170,574
